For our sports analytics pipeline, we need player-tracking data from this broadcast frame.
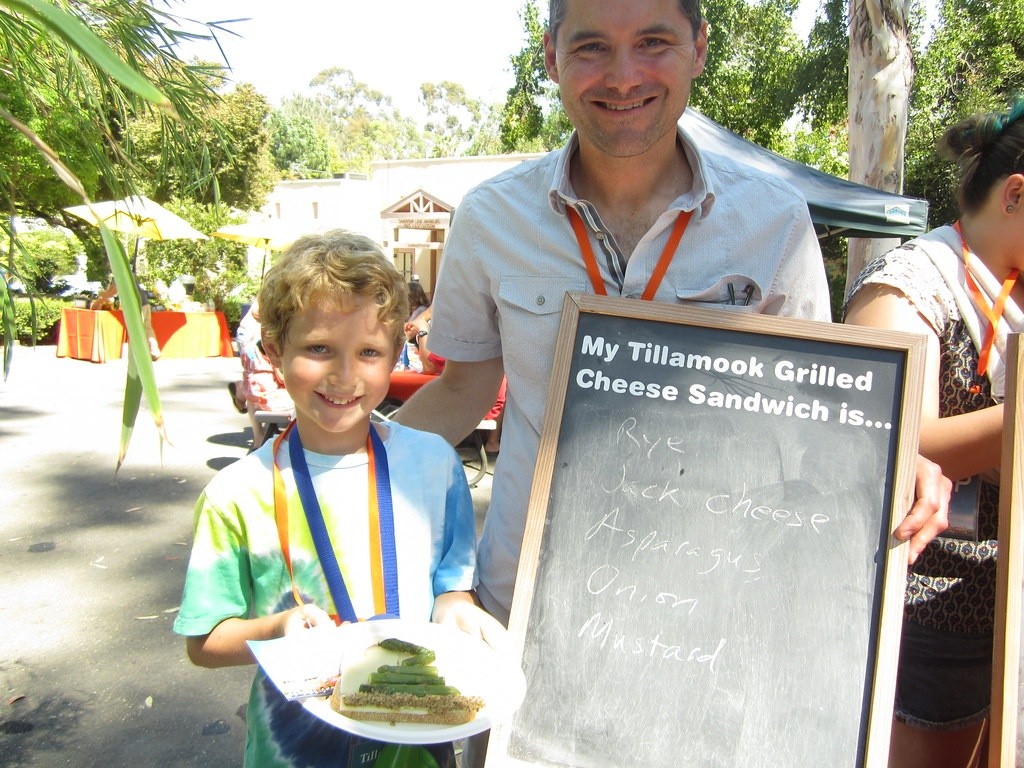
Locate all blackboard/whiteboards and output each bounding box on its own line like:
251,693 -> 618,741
483,292 -> 928,768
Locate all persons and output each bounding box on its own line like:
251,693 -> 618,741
406,304 -> 509,443
237,338 -> 300,457
229,293 -> 273,412
93,251 -> 163,361
172,231 -> 480,767
837,99 -> 1024,763
376,3 -> 952,629
398,280 -> 430,368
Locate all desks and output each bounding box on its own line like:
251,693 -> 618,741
383,369 -> 498,491
56,307 -> 236,363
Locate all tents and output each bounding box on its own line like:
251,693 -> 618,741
682,105 -> 928,326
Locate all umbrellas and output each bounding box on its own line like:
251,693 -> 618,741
59,195 -> 211,306
214,213 -> 296,296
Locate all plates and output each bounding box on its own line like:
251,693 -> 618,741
297,618 -> 528,746
